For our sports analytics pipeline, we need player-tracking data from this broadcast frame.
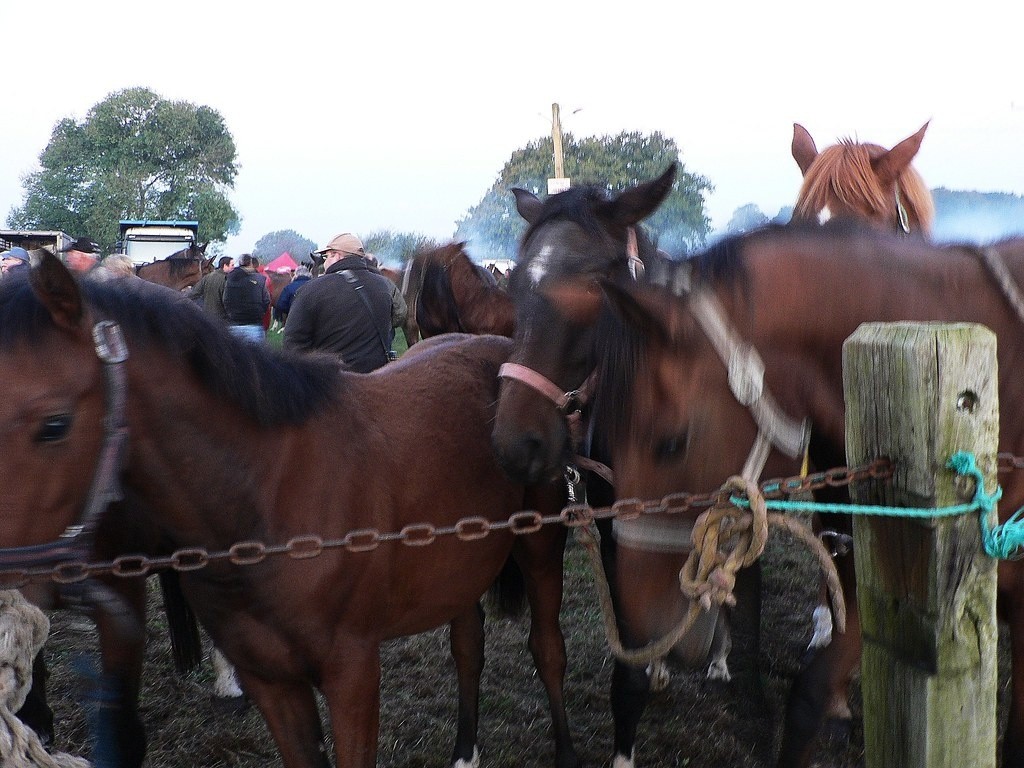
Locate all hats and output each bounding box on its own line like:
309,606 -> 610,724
73,237 -> 101,254
313,232 -> 366,257
1,247 -> 30,261
58,242 -> 77,253
294,266 -> 309,276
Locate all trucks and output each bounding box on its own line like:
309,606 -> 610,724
115,219 -> 203,276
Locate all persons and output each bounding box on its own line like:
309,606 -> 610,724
183,254 -> 276,344
276,230 -> 407,375
0,238 -> 137,279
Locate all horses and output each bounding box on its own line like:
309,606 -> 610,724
0,118 -> 1024,768
